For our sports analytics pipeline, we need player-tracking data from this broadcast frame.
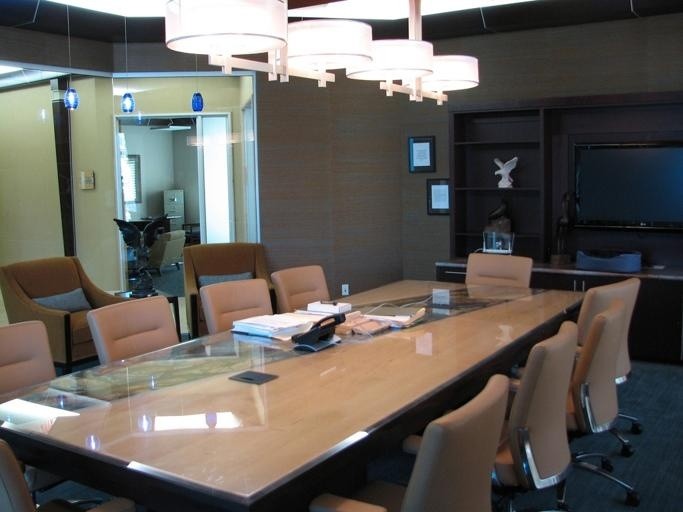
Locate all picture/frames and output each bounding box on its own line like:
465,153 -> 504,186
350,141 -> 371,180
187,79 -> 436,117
407,136 -> 436,172
426,178 -> 449,216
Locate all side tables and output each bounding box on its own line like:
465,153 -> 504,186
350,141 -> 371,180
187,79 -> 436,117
113,287 -> 183,343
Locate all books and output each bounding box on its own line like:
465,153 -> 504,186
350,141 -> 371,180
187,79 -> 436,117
231,301 -> 426,342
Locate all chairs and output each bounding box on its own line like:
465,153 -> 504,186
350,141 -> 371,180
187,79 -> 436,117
492,321 -> 641,511
1,437 -> 135,512
577,278 -> 642,384
199,280 -> 273,335
465,253 -> 533,288
270,266 -> 330,312
86,296 -> 180,365
0,320 -> 56,392
308,374 -> 508,512
566,303 -> 644,457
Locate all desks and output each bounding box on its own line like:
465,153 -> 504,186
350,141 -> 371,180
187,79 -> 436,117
0,279 -> 585,505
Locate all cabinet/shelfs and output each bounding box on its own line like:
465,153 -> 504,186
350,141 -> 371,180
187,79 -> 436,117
548,93 -> 683,266
448,107 -> 545,264
435,260 -> 683,365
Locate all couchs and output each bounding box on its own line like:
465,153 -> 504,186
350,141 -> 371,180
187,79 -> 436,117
148,230 -> 186,276
180,242 -> 278,336
0,256 -> 135,373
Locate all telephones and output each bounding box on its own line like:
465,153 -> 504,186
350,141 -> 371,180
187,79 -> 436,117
291,312 -> 346,352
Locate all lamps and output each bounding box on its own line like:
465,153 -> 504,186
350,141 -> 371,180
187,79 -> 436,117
64,88 -> 79,111
191,93 -> 204,113
400,56 -> 480,105
346,41 -> 434,97
165,0 -> 286,74
120,94 -> 134,113
268,19 -> 372,80
